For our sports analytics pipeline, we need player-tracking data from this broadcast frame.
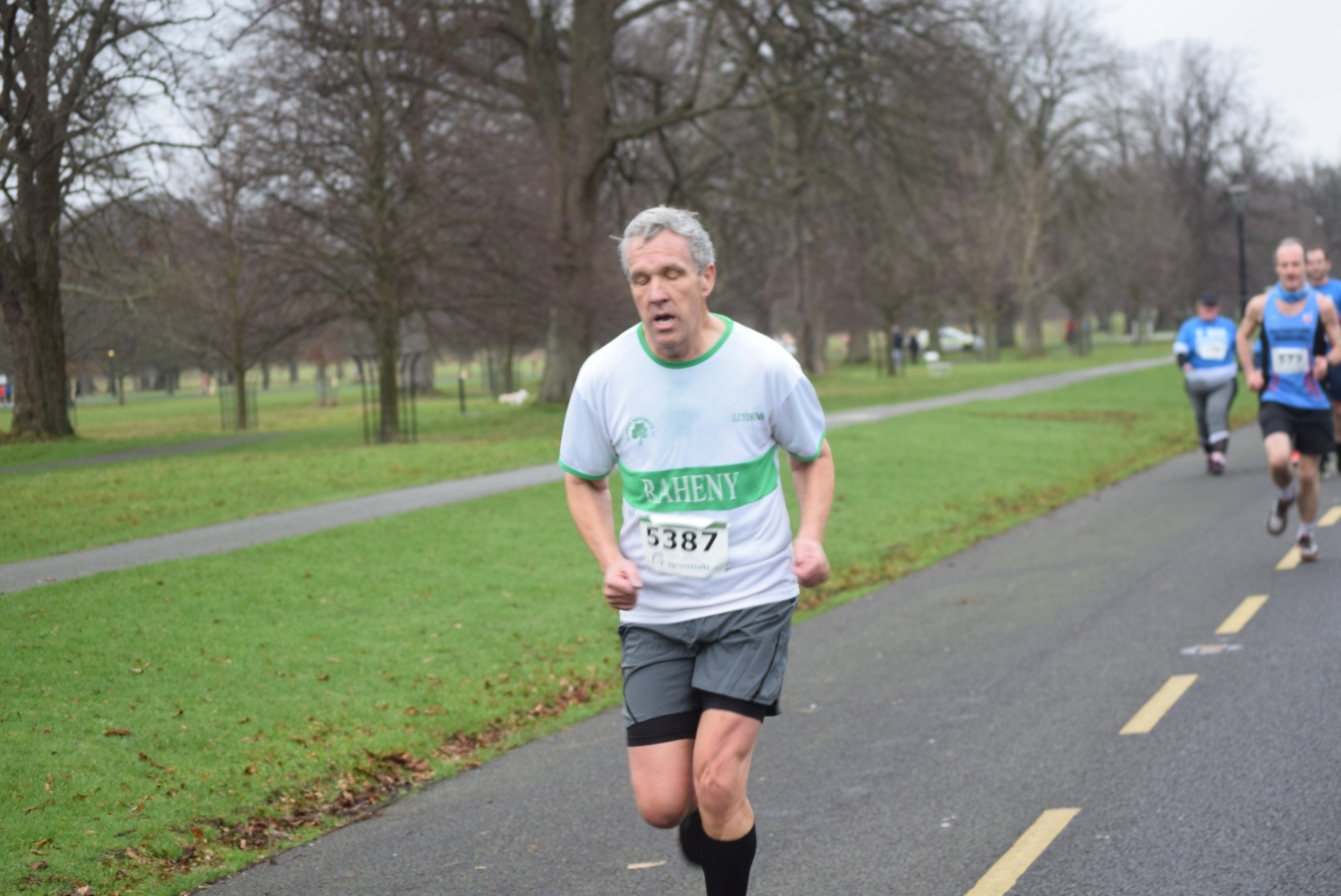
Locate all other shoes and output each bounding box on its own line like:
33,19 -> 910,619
679,808 -> 702,865
1290,448 -> 1301,464
1207,455 -> 1225,473
1317,452 -> 1335,480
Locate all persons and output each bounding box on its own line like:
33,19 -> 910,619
0,383 -> 11,404
1066,315 -> 1090,355
1173,293 -> 1239,476
890,325 -> 903,374
1236,237 -> 1341,560
906,327 -> 920,363
557,204 -> 835,895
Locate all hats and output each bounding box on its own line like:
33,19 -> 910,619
1196,291 -> 1220,306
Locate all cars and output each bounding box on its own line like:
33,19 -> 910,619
914,327 -> 982,353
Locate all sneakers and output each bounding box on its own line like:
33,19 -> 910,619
1296,529 -> 1319,561
1267,487 -> 1296,535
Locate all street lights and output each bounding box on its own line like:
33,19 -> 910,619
1227,173 -> 1250,320
106,349 -> 116,395
